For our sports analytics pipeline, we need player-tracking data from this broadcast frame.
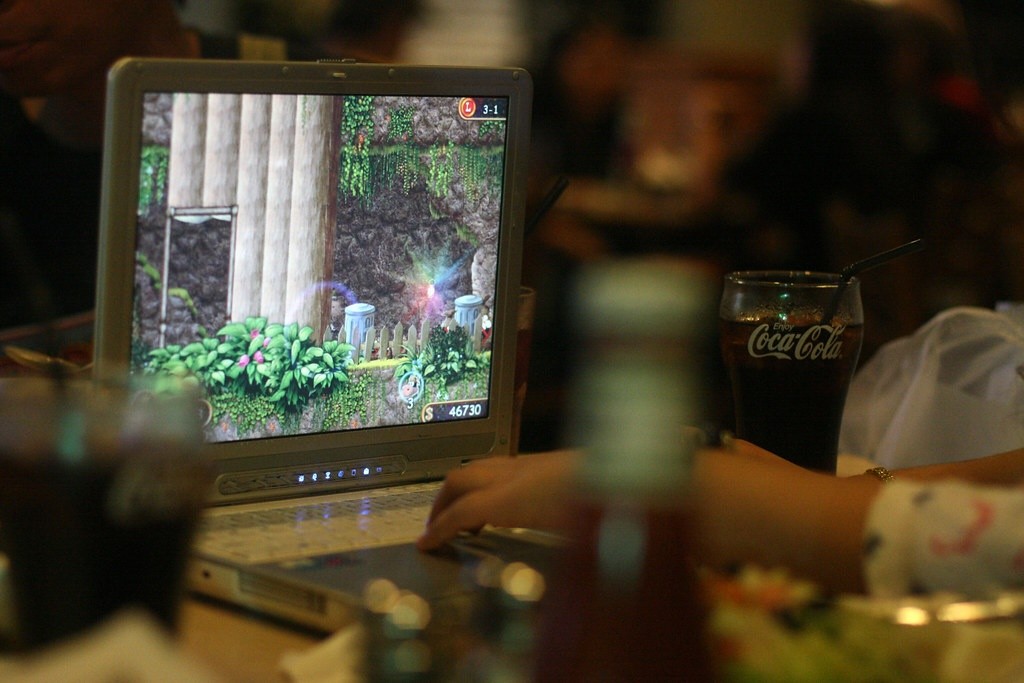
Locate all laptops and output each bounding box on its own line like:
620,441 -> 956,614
88,56 -> 576,635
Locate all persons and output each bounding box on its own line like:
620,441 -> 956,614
0,0 -> 1024,324
419,415 -> 1024,621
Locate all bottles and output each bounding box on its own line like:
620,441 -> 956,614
536,254 -> 711,683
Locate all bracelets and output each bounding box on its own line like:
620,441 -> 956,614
867,467 -> 896,485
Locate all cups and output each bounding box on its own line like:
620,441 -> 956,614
0,376 -> 213,657
509,288 -> 533,457
719,270 -> 864,477
